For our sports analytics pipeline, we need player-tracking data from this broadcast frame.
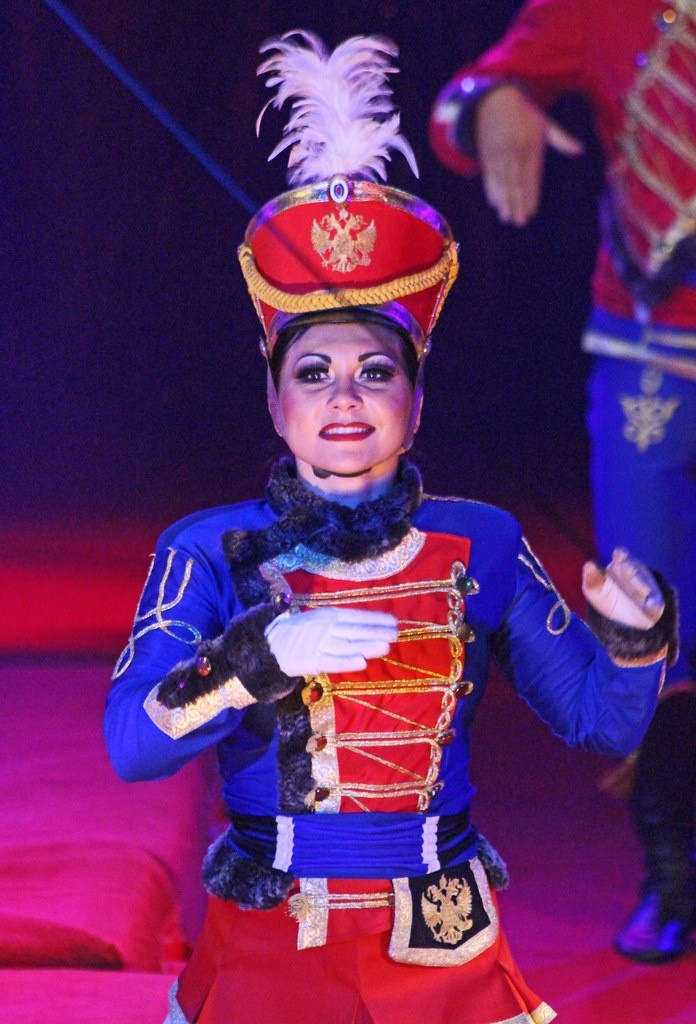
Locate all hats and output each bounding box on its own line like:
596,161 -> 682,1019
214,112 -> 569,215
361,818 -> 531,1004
238,30 -> 460,363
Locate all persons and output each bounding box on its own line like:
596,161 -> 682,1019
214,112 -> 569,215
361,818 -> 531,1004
101,31 -> 679,1024
433,0 -> 696,965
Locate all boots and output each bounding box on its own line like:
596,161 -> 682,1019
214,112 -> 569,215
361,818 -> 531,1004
613,684 -> 696,964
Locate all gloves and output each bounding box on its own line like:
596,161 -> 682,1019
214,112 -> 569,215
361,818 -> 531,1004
581,547 -> 665,631
267,606 -> 398,677
